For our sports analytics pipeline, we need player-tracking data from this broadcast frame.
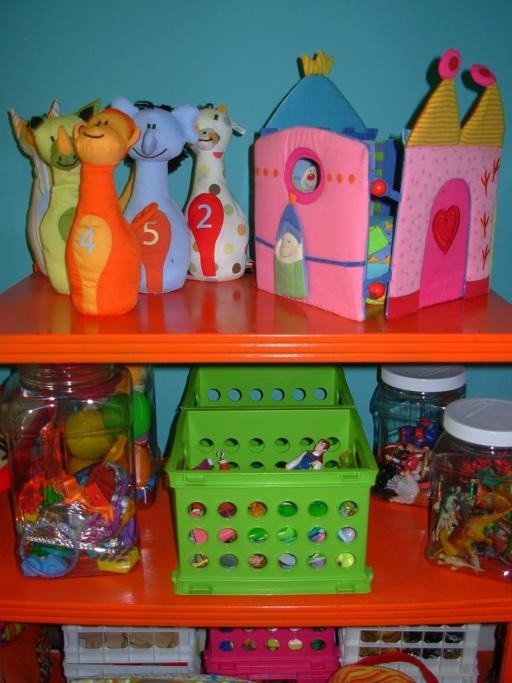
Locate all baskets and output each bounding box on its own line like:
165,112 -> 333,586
165,366 -> 380,597
63,624 -> 483,682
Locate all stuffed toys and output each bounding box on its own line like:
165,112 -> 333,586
5,91 -> 253,317
5,92 -> 253,316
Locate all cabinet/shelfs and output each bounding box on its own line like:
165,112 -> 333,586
0,272 -> 512,683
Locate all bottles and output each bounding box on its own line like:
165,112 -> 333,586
0,362 -> 159,578
366,364 -> 468,509
425,397 -> 512,583
